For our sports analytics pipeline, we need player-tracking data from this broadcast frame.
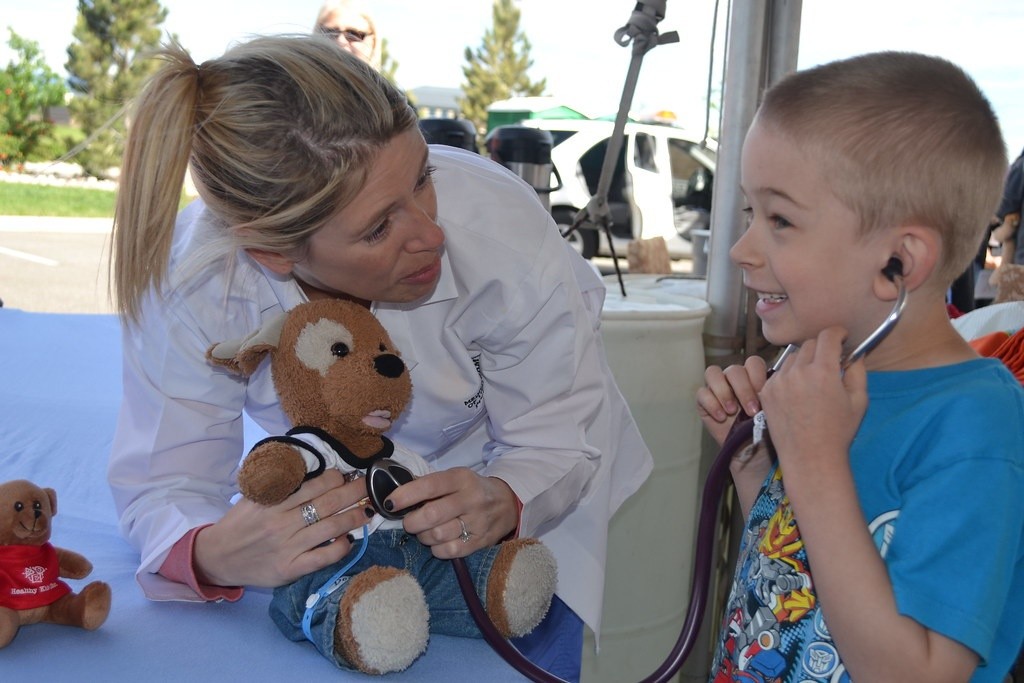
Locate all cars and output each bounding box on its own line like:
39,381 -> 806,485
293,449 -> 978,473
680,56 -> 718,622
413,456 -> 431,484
524,116 -> 722,259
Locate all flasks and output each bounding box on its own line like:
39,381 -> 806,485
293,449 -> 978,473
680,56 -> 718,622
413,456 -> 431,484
418,119 -> 480,155
484,125 -> 563,219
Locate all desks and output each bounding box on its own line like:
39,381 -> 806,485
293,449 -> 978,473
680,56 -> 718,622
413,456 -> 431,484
0,310 -> 531,683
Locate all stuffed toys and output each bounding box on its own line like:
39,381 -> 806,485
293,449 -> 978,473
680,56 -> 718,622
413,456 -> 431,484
0,480 -> 111,649
206,299 -> 557,675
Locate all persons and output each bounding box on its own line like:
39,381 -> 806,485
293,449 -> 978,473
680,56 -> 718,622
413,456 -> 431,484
314,0 -> 381,67
945,149 -> 1024,314
696,51 -> 1024,683
107,33 -> 653,682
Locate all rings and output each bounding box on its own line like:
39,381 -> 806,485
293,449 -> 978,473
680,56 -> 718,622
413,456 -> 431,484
458,517 -> 471,543
301,502 -> 319,526
